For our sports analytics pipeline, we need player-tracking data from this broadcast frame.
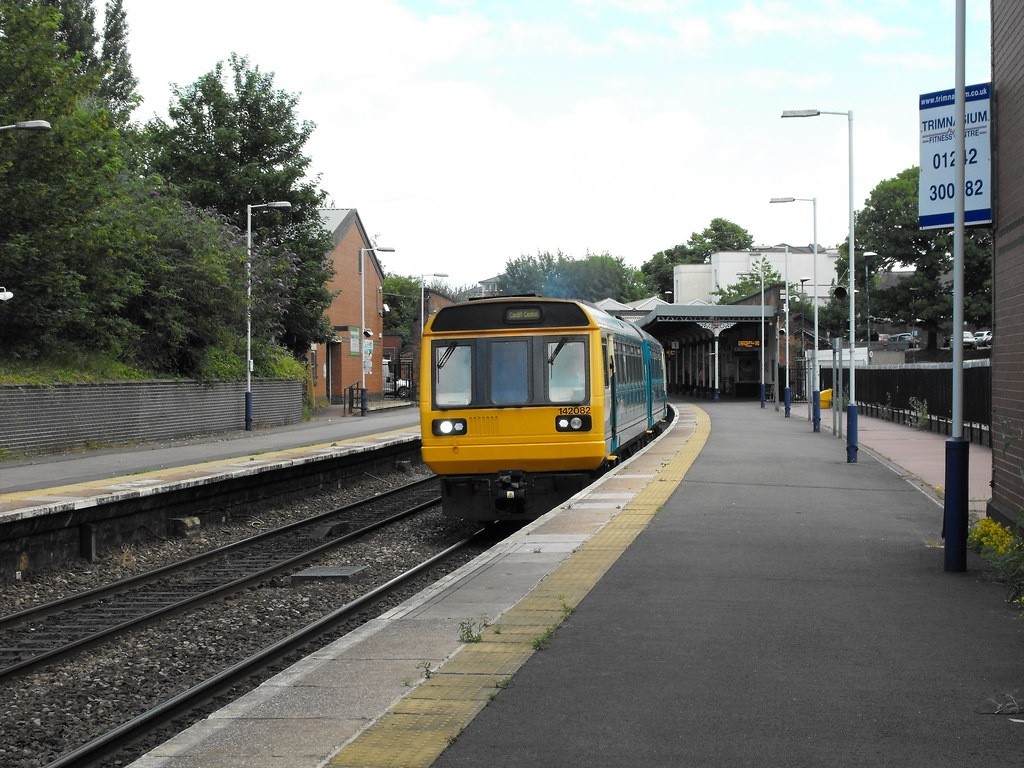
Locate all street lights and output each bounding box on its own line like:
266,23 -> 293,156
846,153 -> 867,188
421,273 -> 449,335
245,201 -> 292,431
781,110 -> 858,463
769,198 -> 821,433
361,247 -> 395,416
751,246 -> 791,418
736,271 -> 767,408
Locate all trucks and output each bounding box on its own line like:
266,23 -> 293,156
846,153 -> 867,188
382,359 -> 416,399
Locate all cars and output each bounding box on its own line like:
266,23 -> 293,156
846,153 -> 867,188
949,331 -> 977,350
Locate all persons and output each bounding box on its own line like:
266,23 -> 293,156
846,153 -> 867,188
698,367 -> 703,387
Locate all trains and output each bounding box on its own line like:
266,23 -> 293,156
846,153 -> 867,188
419,293 -> 669,521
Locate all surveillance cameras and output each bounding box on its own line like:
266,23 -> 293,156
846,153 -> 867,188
795,298 -> 800,303
383,304 -> 390,313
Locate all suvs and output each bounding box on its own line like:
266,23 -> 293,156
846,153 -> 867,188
879,333 -> 920,348
974,331 -> 992,347
